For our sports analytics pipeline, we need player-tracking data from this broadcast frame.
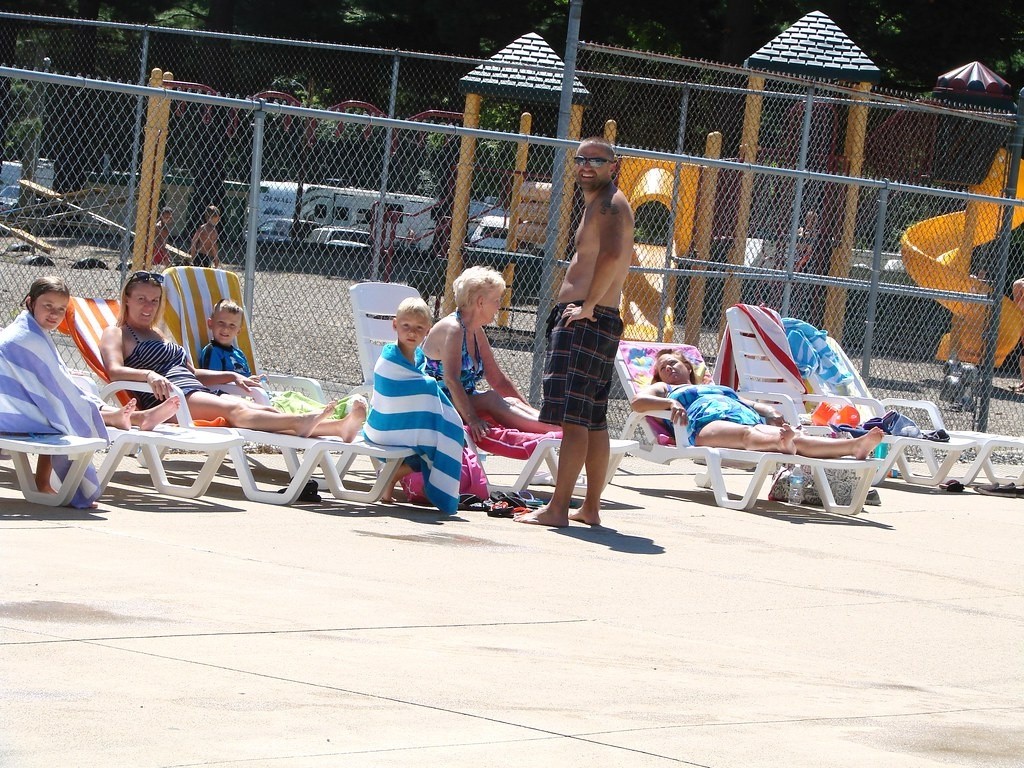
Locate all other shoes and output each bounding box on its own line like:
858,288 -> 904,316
865,489 -> 880,506
531,472 -> 588,487
279,476 -> 323,504
940,479 -> 962,491
974,482 -> 1023,498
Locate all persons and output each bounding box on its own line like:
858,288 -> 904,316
198,299 -> 269,385
99,271 -> 367,443
114,207 -> 172,270
1012,278 -> 1024,392
796,212 -> 818,255
422,266 -> 564,442
0,276 -> 110,508
83,390 -> 180,431
512,136 -> 636,528
361,297 -> 465,515
630,349 -> 884,460
191,206 -> 220,269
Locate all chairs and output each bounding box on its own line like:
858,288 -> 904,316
0,268 -> 1024,514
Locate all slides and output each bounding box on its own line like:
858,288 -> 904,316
615,235 -> 685,342
900,146 -> 1024,368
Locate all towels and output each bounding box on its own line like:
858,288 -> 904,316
361,343 -> 464,516
0,310 -> 110,510
781,317 -> 856,387
712,302 -> 809,405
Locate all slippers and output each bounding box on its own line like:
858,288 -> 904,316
456,490 -> 579,518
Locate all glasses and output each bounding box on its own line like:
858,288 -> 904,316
125,271 -> 165,288
573,155 -> 615,168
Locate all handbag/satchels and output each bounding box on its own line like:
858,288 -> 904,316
771,461 -> 866,512
